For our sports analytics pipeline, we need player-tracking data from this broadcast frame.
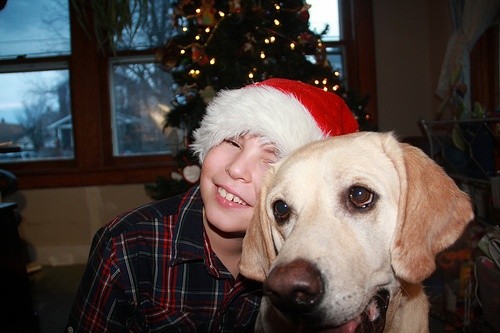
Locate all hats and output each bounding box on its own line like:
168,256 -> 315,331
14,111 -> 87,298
188,77 -> 360,172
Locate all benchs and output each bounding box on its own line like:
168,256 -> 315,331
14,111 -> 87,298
3,183 -> 155,302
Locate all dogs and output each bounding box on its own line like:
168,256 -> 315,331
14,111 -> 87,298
236,130 -> 476,331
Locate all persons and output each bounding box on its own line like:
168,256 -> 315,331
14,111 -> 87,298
65,78 -> 359,333
